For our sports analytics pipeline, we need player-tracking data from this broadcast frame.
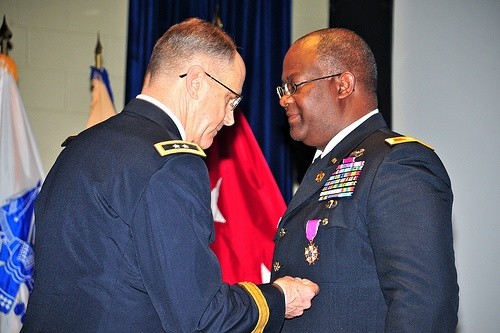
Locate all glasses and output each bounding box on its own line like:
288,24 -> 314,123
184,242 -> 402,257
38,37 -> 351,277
276,73 -> 356,99
178,71 -> 243,112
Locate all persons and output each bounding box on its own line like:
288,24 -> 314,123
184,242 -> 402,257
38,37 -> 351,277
269,26 -> 462,333
20,18 -> 320,333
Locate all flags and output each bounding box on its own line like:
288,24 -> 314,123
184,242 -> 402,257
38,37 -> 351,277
87,61 -> 119,131
206,104 -> 288,289
0,53 -> 47,333
